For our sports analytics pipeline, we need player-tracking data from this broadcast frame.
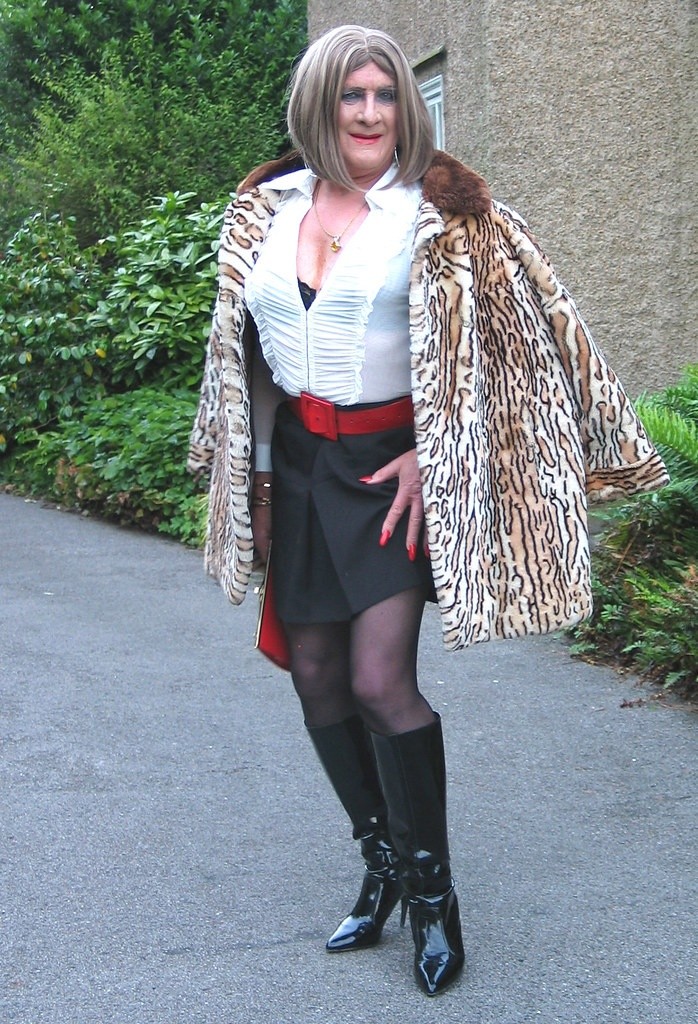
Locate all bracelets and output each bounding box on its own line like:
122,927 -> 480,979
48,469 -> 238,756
251,496 -> 272,506
253,482 -> 271,487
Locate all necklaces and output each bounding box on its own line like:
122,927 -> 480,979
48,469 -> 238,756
314,180 -> 366,252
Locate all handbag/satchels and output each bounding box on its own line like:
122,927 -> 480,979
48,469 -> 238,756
254,539 -> 292,672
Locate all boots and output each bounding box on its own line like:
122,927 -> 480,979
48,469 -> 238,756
370,711 -> 466,997
304,711 -> 409,956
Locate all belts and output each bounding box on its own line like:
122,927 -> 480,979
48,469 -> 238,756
285,391 -> 414,440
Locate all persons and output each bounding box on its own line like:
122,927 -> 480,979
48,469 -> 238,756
186,25 -> 670,997
